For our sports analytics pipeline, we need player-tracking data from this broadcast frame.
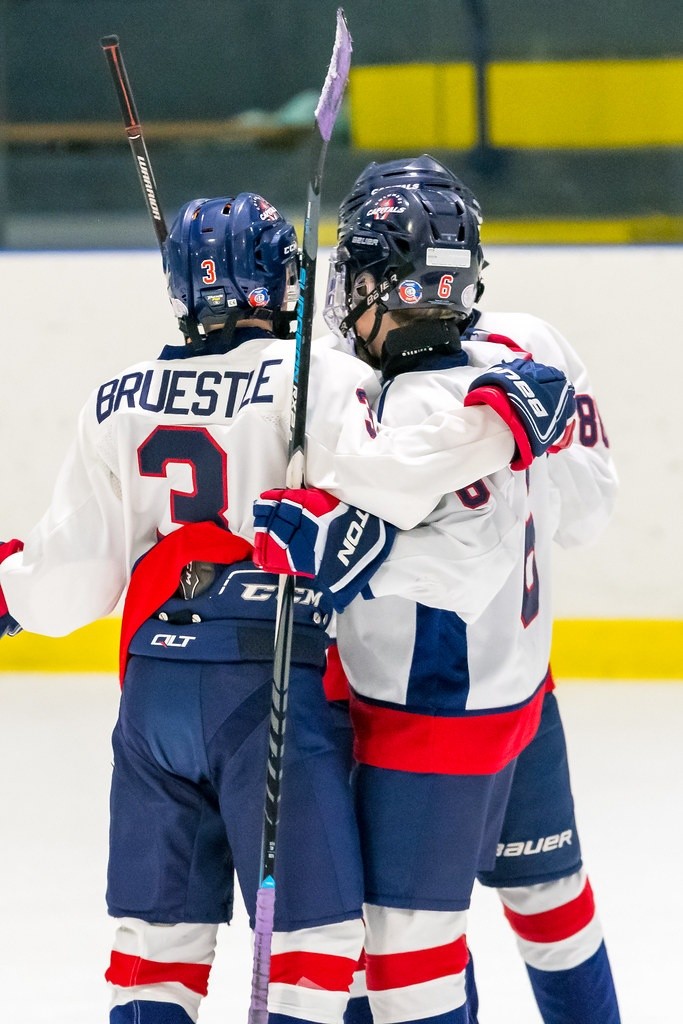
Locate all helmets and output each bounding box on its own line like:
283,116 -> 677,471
162,192 -> 298,336
336,154 -> 476,239
334,186 -> 489,317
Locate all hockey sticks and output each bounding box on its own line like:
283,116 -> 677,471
101,31 -> 169,254
247,5 -> 357,1024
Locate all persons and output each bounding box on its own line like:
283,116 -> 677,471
223,148 -> 636,1024
0,191 -> 600,1024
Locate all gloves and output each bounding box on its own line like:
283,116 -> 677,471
464,357 -> 577,471
252,488 -> 395,614
0,539 -> 23,637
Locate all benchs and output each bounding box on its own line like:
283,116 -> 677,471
292,56 -> 683,244
